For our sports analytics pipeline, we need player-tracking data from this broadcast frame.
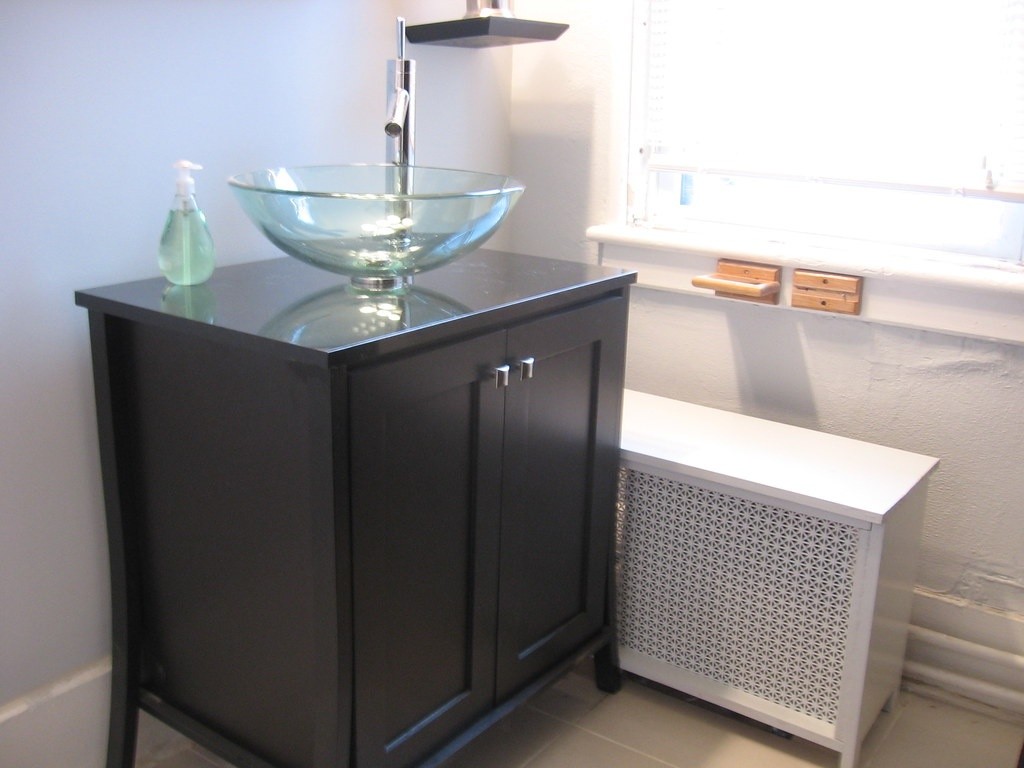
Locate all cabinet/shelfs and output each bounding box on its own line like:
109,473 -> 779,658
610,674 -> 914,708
615,386 -> 944,768
76,227 -> 639,768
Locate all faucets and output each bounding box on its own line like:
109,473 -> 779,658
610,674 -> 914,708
384,17 -> 416,287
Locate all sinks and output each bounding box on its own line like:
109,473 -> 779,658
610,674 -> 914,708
227,163 -> 527,278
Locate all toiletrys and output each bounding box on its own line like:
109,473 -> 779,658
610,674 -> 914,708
156,160 -> 216,286
